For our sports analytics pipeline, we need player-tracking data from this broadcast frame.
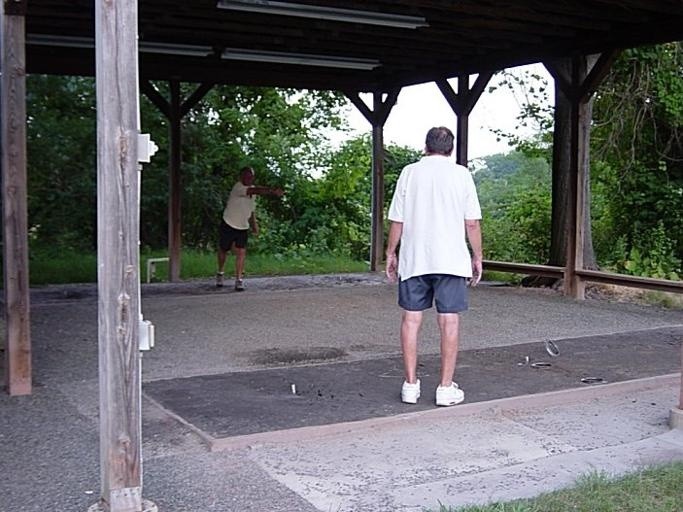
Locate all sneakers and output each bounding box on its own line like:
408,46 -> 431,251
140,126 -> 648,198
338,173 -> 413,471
400,377 -> 422,404
433,380 -> 466,407
214,272 -> 224,287
232,278 -> 244,291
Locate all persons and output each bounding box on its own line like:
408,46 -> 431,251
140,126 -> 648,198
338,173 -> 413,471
386,127 -> 486,406
216,167 -> 284,291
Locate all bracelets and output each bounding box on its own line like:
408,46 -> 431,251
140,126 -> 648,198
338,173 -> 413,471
268,189 -> 271,194
386,253 -> 397,259
